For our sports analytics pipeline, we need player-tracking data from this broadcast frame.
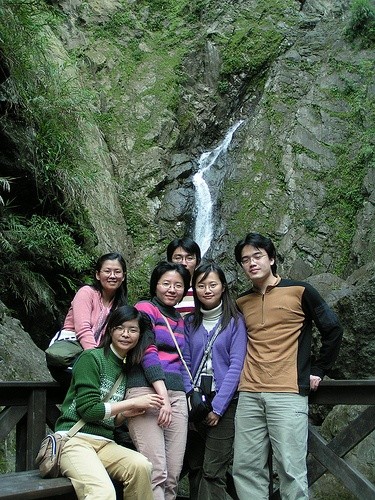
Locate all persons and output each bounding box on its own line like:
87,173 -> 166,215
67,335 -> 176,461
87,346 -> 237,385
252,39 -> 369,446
48,236 -> 246,500
234,233 -> 344,500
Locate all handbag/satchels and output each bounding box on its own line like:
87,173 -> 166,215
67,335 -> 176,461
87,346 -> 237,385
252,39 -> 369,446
185,386 -> 214,422
44,338 -> 83,374
34,432 -> 64,478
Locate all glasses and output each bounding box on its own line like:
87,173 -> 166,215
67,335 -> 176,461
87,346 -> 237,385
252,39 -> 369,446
157,282 -> 183,290
171,255 -> 196,262
240,253 -> 267,265
100,269 -> 124,278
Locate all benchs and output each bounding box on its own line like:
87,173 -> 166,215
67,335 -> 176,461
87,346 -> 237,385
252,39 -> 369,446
0,381 -> 124,500
